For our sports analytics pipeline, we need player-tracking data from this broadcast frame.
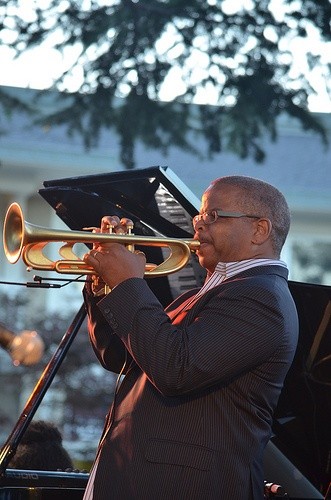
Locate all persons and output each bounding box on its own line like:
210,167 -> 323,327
81,175 -> 300,500
7,419 -> 72,473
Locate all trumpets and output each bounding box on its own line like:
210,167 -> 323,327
0,202 -> 203,280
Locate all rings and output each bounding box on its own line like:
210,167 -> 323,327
135,251 -> 146,255
93,250 -> 104,258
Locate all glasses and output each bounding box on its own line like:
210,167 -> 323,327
192,208 -> 262,225
